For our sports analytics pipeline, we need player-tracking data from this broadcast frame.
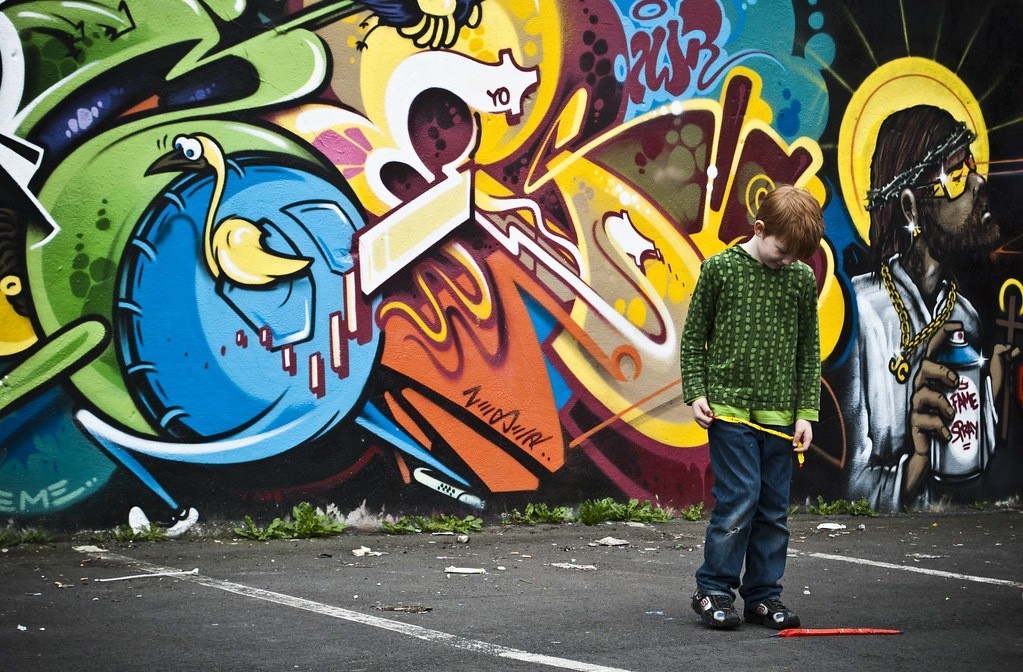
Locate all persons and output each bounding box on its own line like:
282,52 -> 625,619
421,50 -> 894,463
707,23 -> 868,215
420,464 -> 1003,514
681,185 -> 823,631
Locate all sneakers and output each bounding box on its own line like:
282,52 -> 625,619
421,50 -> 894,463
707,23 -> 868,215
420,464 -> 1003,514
691,587 -> 742,628
744,596 -> 800,629
